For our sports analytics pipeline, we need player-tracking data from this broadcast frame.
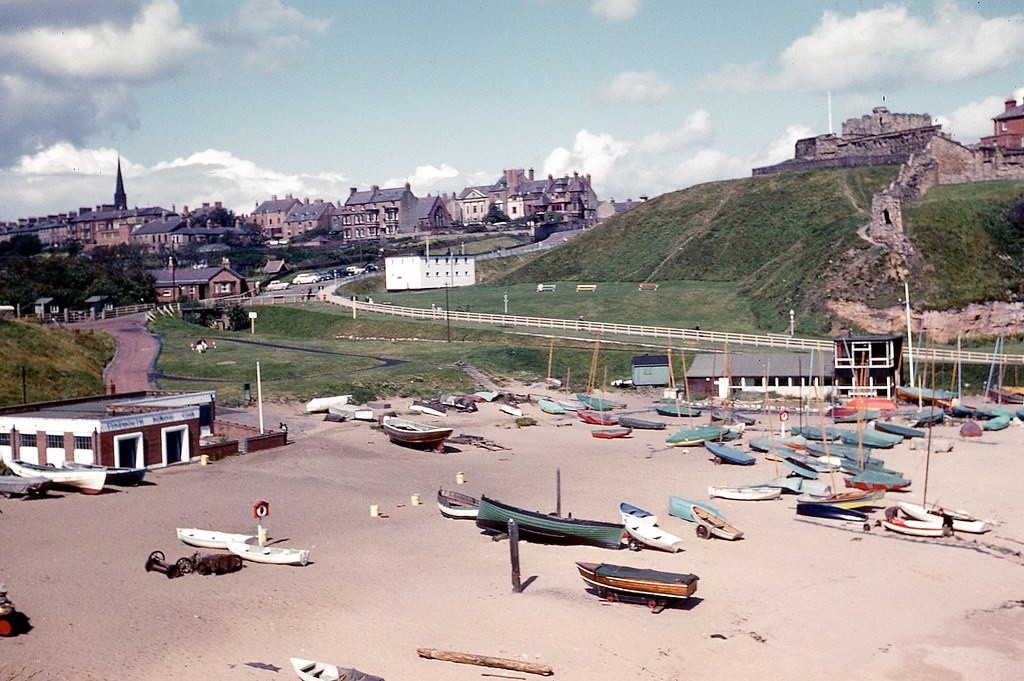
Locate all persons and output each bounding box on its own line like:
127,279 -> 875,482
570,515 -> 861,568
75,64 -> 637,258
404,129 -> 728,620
578,316 -> 584,327
937,508 -> 955,536
693,322 -> 703,337
431,303 -> 442,316
197,337 -> 208,353
365,296 -> 374,304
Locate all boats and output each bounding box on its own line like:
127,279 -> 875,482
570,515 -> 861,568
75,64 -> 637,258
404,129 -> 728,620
575,559 -> 700,607
176,528 -> 257,549
382,415 -> 454,448
620,502 -> 681,553
656,343 -> 756,465
669,496 -> 744,541
306,396 -> 380,422
437,487 -> 481,520
749,281 -> 1024,536
290,658 -> 387,681
226,540 -> 312,566
62,460 -> 147,487
476,493 -> 626,550
440,331 -> 666,439
0,456 -> 107,495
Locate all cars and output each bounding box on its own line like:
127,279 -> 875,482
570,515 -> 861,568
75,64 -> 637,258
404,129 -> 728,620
266,280 -> 290,290
293,265 -> 366,285
365,264 -> 378,271
